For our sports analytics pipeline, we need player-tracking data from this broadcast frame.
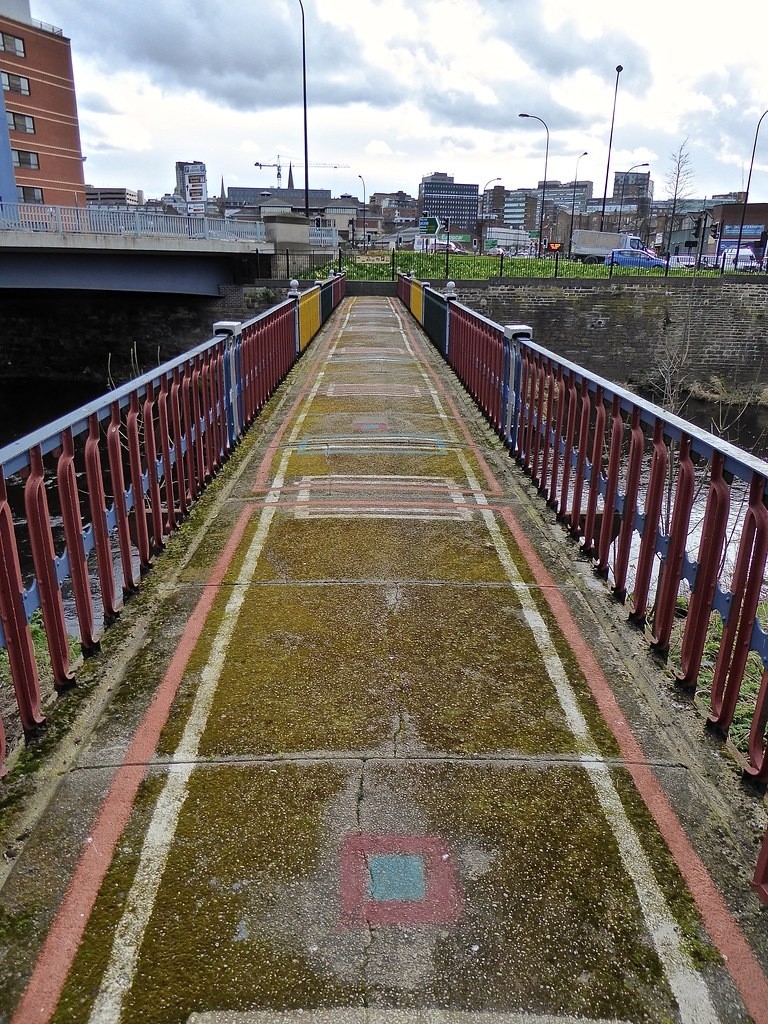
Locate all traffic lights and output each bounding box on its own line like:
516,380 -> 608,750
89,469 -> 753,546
422,211 -> 429,217
691,218 -> 701,238
543,238 -> 548,248
710,222 -> 719,240
398,237 -> 403,247
367,234 -> 372,245
442,217 -> 450,232
473,239 -> 478,249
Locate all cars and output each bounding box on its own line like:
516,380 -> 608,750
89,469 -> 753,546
604,247 -> 768,274
487,247 -> 505,258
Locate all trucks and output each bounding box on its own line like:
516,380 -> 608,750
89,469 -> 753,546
571,230 -> 657,264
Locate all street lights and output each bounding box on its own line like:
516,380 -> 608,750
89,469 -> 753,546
568,152 -> 588,259
518,113 -> 549,258
479,178 -> 502,256
618,163 -> 650,234
358,175 -> 366,251
599,66 -> 624,232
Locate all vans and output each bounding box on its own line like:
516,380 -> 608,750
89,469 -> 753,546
431,242 -> 456,254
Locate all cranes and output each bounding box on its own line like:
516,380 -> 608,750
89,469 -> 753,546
253,154 -> 350,189
